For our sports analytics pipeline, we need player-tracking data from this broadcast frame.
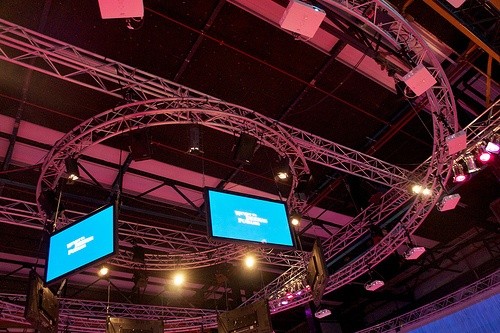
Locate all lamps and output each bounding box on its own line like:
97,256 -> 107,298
450,128 -> 499,183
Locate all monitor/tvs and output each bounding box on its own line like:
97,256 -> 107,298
202,186 -> 297,252
217,298 -> 274,333
304,237 -> 327,308
23,269 -> 60,333
106,316 -> 164,333
44,199 -> 119,288
